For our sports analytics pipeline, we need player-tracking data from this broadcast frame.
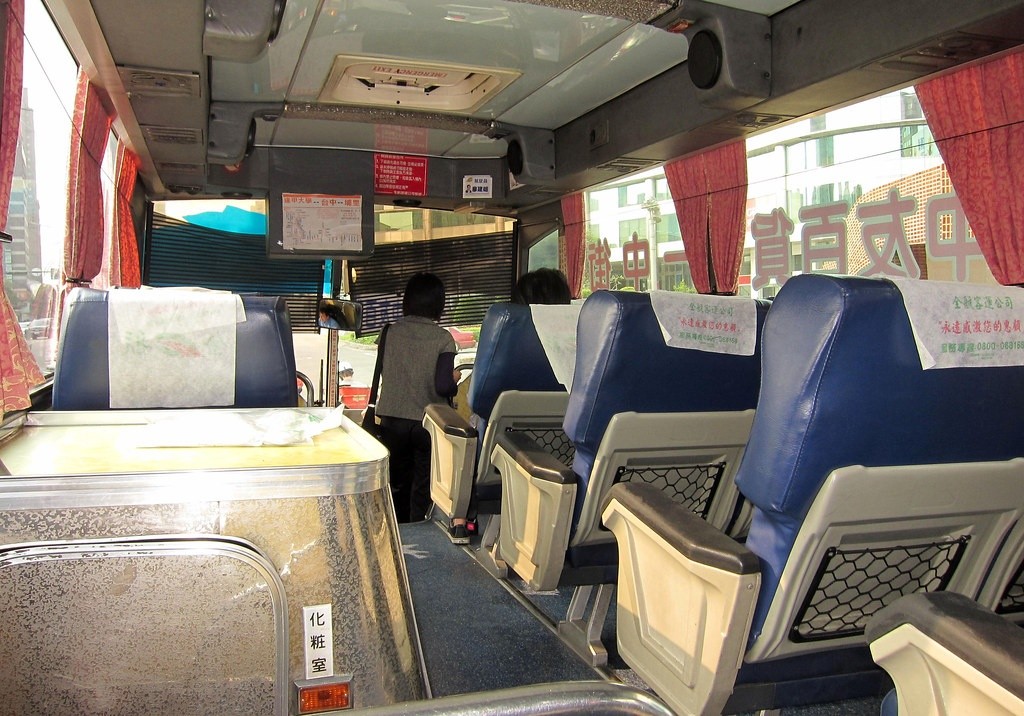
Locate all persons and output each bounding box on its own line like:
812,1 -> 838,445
319,306 -> 340,329
447,268 -> 573,544
378,272 -> 462,522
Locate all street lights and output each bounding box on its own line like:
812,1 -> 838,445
641,195 -> 662,290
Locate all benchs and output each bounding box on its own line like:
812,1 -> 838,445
420,274 -> 1024,715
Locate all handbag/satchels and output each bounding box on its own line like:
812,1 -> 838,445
360,406 -> 385,441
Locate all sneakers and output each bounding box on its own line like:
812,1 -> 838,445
445,517 -> 471,544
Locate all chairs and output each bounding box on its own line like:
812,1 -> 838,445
51,286 -> 315,412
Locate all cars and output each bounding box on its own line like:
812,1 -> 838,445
443,327 -> 476,353
18,322 -> 31,335
25,317 -> 56,340
